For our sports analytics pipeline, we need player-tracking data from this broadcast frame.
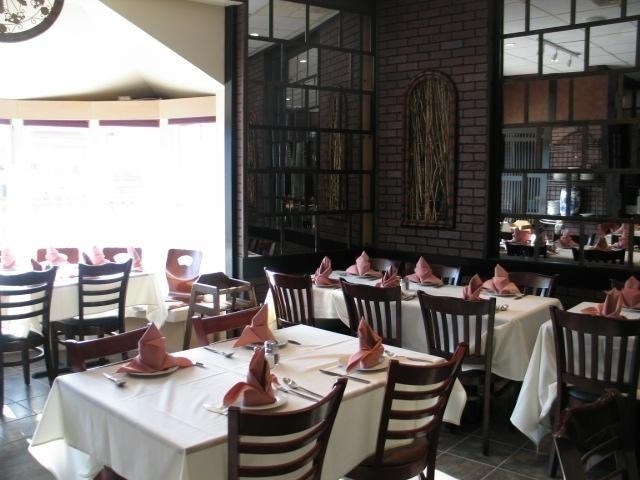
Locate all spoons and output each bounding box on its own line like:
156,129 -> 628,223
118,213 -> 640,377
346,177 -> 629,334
204,347 -> 235,358
283,378 -> 325,399
102,371 -> 126,386
385,351 -> 434,363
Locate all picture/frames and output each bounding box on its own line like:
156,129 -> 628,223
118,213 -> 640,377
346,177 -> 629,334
400,70 -> 460,231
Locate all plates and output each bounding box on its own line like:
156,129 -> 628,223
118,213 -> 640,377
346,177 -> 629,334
337,350 -> 390,371
487,288 -> 521,296
310,275 -> 343,288
580,173 -> 595,180
547,200 -> 560,215
347,269 -> 378,279
552,173 -> 567,180
568,166 -> 580,170
580,212 -> 595,217
246,333 -> 287,348
407,276 -> 443,287
222,382 -> 288,410
621,306 -> 639,312
122,360 -> 178,377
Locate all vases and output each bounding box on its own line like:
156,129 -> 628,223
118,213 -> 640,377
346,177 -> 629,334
547,199 -> 560,217
581,173 -> 596,181
568,165 -> 583,182
554,172 -> 568,182
560,188 -> 584,217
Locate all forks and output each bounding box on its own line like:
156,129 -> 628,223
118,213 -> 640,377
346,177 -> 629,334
273,381 -> 319,403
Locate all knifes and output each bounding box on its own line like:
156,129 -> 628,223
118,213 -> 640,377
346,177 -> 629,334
320,369 -> 370,386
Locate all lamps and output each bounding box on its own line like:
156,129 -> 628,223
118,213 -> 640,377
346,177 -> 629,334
552,46 -> 576,69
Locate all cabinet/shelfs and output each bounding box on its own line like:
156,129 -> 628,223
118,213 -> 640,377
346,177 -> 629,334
546,125 -> 609,218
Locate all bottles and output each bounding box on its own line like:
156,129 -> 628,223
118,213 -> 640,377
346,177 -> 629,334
262,340 -> 281,370
